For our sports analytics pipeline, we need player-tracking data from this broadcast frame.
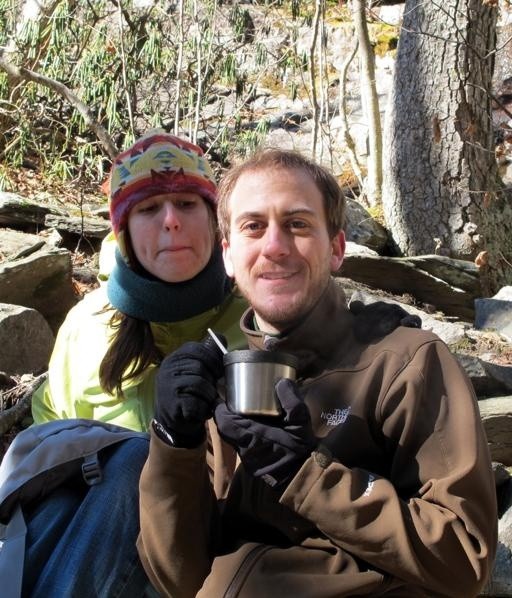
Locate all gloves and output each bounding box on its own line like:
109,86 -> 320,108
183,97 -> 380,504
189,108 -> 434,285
148,333 -> 228,450
214,378 -> 313,493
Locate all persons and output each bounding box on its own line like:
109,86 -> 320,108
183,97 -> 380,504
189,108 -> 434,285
26,128 -> 253,450
135,143 -> 502,596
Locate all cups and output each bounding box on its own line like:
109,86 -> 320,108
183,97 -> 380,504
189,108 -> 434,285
216,350 -> 299,419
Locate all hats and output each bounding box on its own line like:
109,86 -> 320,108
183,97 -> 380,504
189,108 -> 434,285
106,126 -> 222,268
